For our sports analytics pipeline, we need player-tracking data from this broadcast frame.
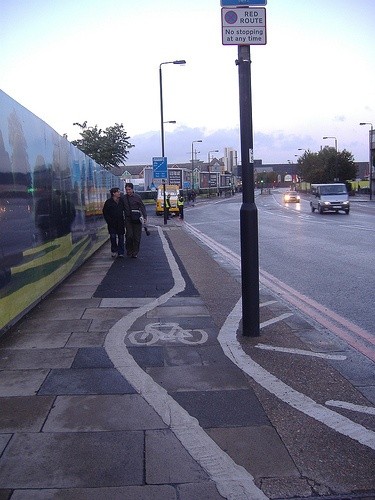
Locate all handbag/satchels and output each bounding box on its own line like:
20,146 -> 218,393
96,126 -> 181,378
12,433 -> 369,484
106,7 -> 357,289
130,213 -> 141,224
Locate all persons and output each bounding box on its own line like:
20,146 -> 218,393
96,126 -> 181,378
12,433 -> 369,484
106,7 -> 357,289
103,187 -> 125,257
184,187 -> 239,207
177,194 -> 184,219
123,183 -> 147,258
165,194 -> 171,220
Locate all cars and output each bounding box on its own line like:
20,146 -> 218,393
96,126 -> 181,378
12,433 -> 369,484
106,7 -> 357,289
283,190 -> 301,203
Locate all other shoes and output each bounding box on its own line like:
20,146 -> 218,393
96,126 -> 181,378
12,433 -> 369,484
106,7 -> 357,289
111,252 -> 116,257
167,217 -> 171,220
178,217 -> 183,220
118,253 -> 124,257
127,250 -> 138,258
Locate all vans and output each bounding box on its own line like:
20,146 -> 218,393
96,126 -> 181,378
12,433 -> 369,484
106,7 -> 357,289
310,183 -> 350,214
154,185 -> 181,216
84,186 -> 108,219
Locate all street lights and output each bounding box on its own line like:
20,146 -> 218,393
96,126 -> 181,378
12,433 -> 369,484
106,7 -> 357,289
208,149 -> 219,198
287,159 -> 293,189
191,140 -> 203,206
360,122 -> 373,202
159,60 -> 187,224
323,136 -> 338,152
